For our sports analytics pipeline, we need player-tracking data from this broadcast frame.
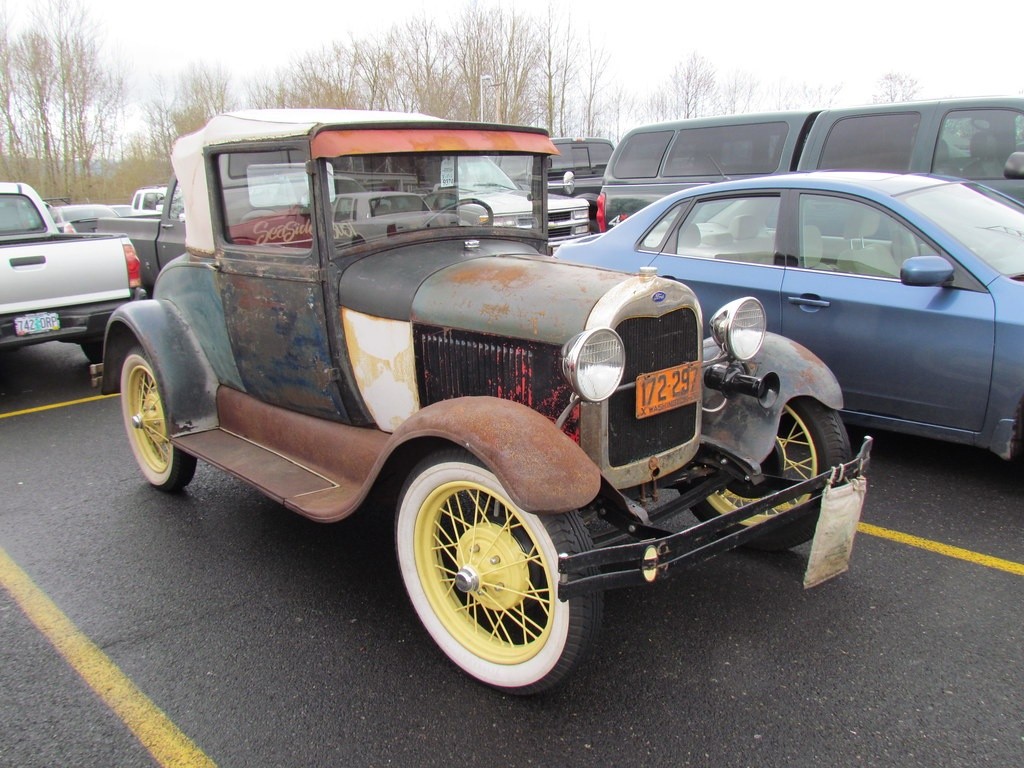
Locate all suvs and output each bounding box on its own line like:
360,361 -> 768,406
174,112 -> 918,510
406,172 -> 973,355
516,131 -> 616,222
439,151 -> 589,249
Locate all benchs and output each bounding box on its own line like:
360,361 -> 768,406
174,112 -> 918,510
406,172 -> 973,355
677,213 -> 774,263
227,205 -> 314,251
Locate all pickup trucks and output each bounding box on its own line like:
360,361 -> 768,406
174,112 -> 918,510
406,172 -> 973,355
0,180 -> 147,364
594,97 -> 1023,241
69,173 -> 185,295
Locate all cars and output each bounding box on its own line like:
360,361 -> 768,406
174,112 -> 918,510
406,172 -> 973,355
41,185 -> 174,223
550,169 -> 1024,478
90,107 -> 875,697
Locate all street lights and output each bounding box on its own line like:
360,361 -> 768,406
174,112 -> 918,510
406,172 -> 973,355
478,74 -> 493,123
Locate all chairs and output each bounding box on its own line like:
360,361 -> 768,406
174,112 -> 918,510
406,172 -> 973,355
935,140 -> 962,178
803,226 -> 833,271
837,207 -> 901,278
970,132 -> 1006,178
